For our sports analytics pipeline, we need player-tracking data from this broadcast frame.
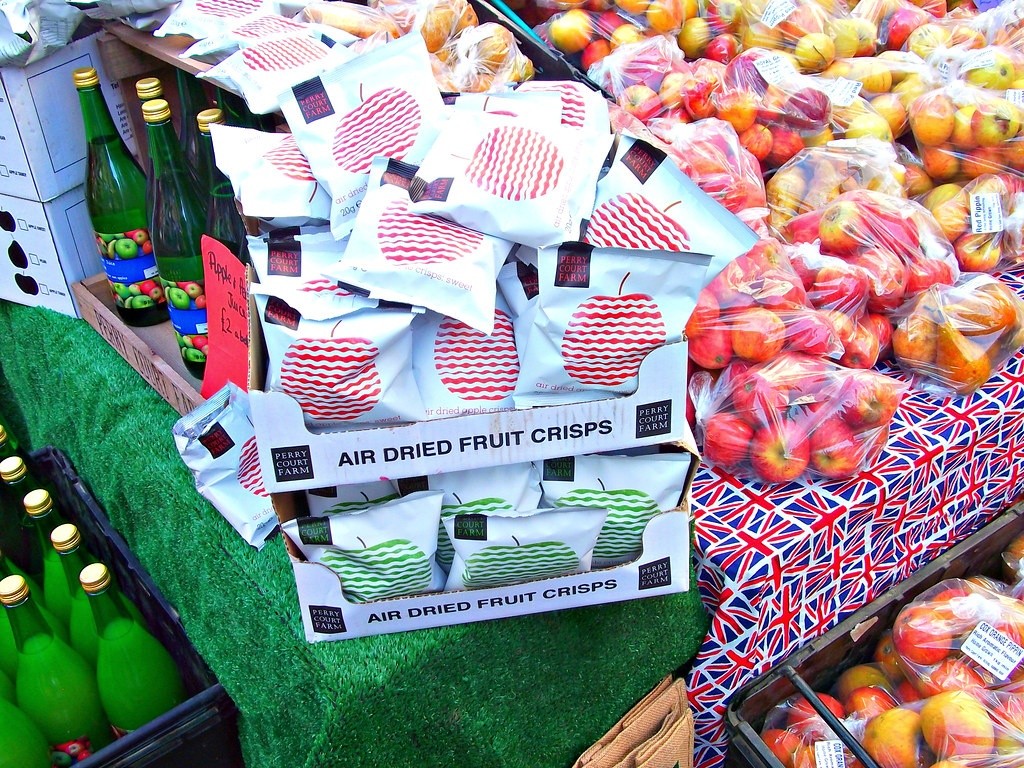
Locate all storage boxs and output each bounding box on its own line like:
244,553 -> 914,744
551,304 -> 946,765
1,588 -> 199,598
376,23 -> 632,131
231,192 -> 704,644
728,493 -> 1023,768
0,445 -> 243,768
0,22 -> 143,320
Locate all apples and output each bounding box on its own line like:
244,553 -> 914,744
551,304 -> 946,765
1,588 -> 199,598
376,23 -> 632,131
758,531 -> 1024,768
304,0 -> 1024,482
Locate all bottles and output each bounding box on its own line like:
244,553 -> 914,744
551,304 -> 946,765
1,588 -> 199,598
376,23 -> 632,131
140,99 -> 214,379
73,67 -> 172,327
195,85 -> 275,269
135,77 -> 209,249
0,422 -> 189,768
175,66 -> 209,182
